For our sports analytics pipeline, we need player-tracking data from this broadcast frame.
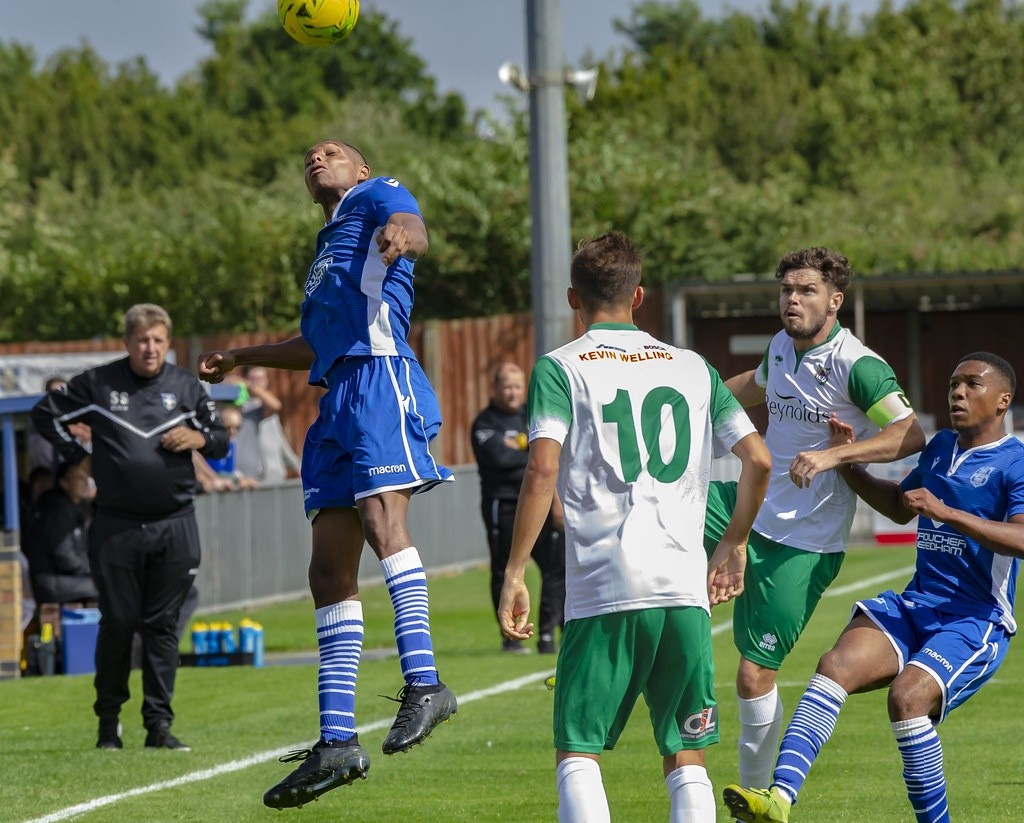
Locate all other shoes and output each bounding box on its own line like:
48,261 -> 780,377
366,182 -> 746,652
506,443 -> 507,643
501,639 -> 530,655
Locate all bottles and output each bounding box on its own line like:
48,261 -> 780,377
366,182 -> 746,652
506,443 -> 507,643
190,618 -> 265,668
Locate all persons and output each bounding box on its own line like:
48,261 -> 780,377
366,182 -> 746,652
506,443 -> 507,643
493,228 -> 775,820
190,404 -> 258,494
46,377 -> 91,452
17,466 -> 52,536
197,365 -> 301,485
192,140 -> 460,807
29,303 -> 228,750
469,359 -> 567,656
702,245 -> 927,796
722,347 -> 1024,821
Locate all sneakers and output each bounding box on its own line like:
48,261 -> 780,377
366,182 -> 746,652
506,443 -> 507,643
143,727 -> 191,752
720,782 -> 792,822
262,733 -> 371,809
377,671 -> 459,755
96,720 -> 123,752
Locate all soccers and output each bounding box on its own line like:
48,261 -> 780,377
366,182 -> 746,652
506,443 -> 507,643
275,0 -> 361,48
